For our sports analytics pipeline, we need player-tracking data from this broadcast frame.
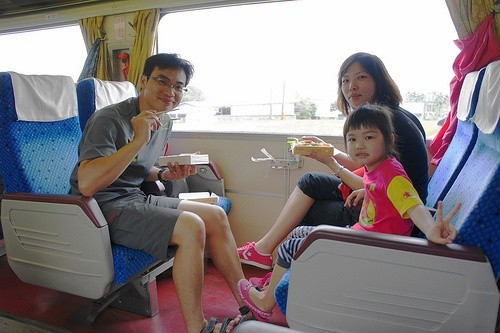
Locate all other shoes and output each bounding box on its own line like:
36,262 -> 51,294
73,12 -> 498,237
238,278 -> 273,322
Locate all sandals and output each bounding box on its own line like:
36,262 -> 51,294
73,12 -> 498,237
238,305 -> 258,321
200,316 -> 235,333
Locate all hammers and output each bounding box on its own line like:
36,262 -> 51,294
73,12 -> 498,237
115,53 -> 129,80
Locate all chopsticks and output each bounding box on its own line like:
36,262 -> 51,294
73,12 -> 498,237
154,108 -> 180,115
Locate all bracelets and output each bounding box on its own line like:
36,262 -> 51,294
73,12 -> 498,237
334,166 -> 344,178
158,169 -> 170,183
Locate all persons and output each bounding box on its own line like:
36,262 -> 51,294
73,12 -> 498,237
69,53 -> 262,333
237,52 -> 429,291
237,104 -> 462,321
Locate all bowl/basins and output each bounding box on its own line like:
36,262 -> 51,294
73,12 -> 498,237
291,142 -> 335,157
158,154 -> 209,167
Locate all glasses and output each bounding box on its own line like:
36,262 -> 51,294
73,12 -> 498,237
148,74 -> 187,93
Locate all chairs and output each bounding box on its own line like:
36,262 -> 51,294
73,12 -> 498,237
0,61 -> 500,333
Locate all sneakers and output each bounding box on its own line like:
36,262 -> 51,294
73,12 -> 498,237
237,241 -> 273,269
249,272 -> 272,289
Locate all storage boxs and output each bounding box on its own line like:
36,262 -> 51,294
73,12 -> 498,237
159,156 -> 210,166
179,192 -> 219,203
294,143 -> 334,156
